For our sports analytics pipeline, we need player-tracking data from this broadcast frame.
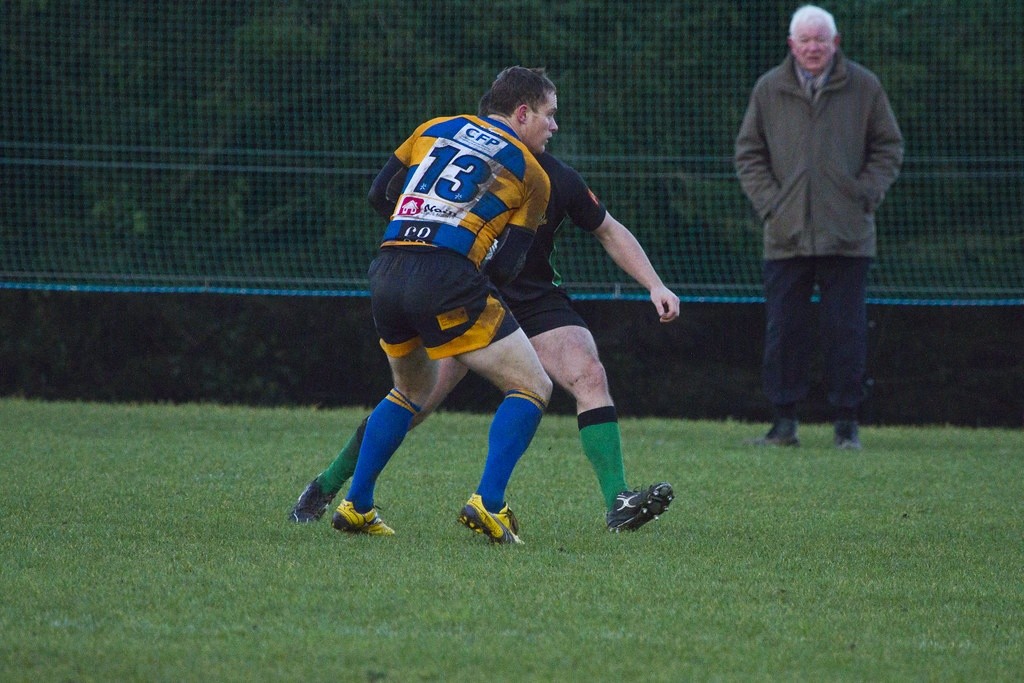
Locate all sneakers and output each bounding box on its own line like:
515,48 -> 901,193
288,474 -> 335,523
331,501 -> 395,536
459,494 -> 523,544
606,482 -> 673,534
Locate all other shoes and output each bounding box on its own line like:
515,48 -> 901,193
744,419 -> 801,446
833,422 -> 862,450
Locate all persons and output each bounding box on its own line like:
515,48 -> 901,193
288,92 -> 680,530
736,5 -> 904,448
332,66 -> 557,545
